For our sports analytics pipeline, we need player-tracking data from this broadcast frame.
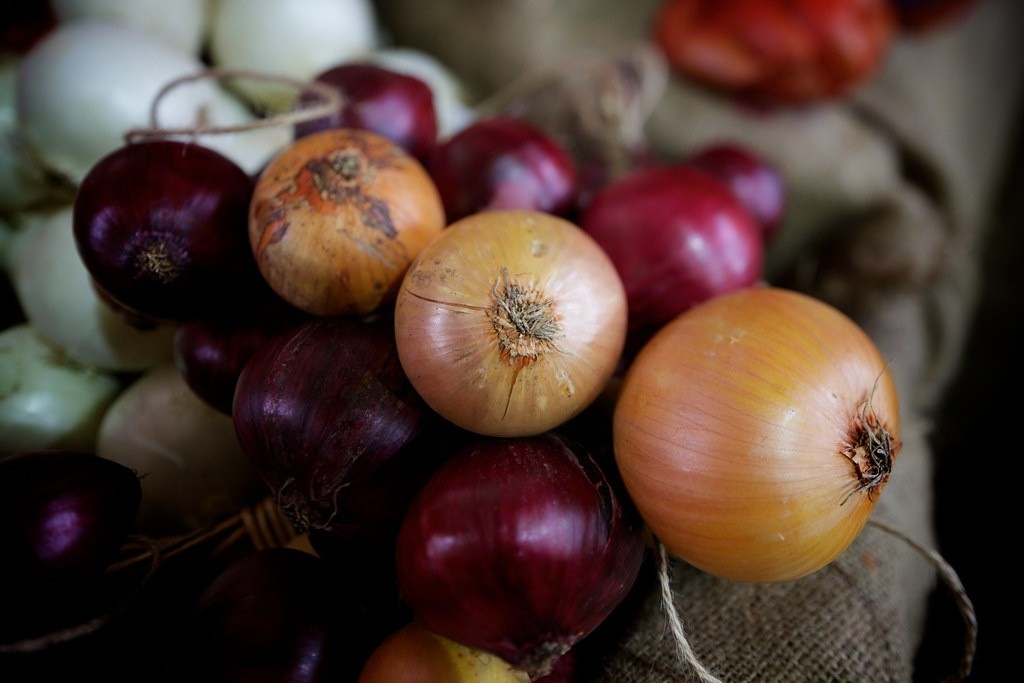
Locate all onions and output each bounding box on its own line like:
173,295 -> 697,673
0,0 -> 900,683
